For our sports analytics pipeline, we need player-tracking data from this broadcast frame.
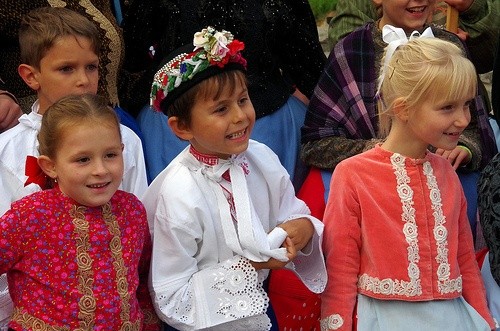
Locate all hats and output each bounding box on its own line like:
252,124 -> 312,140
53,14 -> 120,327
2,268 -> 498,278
149,26 -> 248,114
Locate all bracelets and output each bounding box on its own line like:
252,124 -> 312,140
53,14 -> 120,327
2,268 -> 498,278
456,145 -> 472,163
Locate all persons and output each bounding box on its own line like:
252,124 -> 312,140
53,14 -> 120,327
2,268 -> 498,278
0,0 -> 500,331
